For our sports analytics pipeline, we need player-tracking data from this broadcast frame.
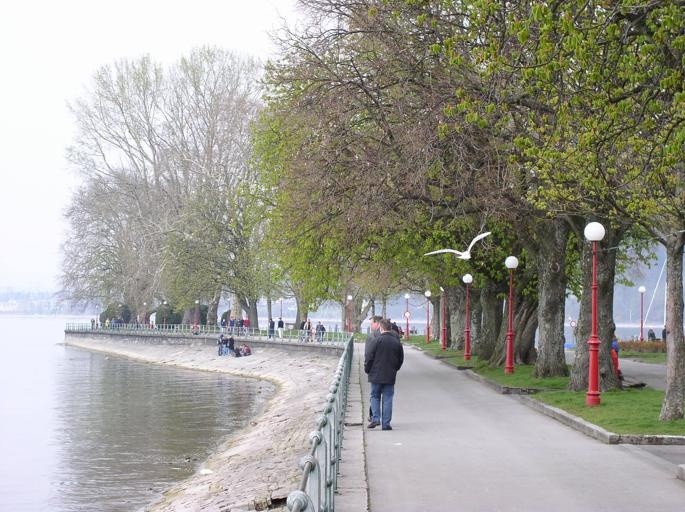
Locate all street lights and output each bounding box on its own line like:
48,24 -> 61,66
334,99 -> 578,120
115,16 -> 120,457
460,273 -> 474,358
143,302 -> 147,326
638,284 -> 646,340
346,295 -> 353,334
424,289 -> 433,342
93,303 -> 99,327
504,255 -> 520,372
163,301 -> 169,329
404,294 -> 411,338
438,284 -> 449,351
193,299 -> 200,332
583,220 -> 603,407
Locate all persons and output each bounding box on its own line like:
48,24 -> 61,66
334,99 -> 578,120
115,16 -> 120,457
364,315 -> 400,421
387,318 -> 403,337
648,325 -> 666,341
90,316 -> 116,331
216,316 -> 326,357
364,319 -> 404,430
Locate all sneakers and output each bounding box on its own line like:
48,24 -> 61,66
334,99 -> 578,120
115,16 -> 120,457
367,417 -> 392,430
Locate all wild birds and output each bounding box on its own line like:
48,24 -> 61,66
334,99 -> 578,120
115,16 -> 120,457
423,231 -> 493,262
